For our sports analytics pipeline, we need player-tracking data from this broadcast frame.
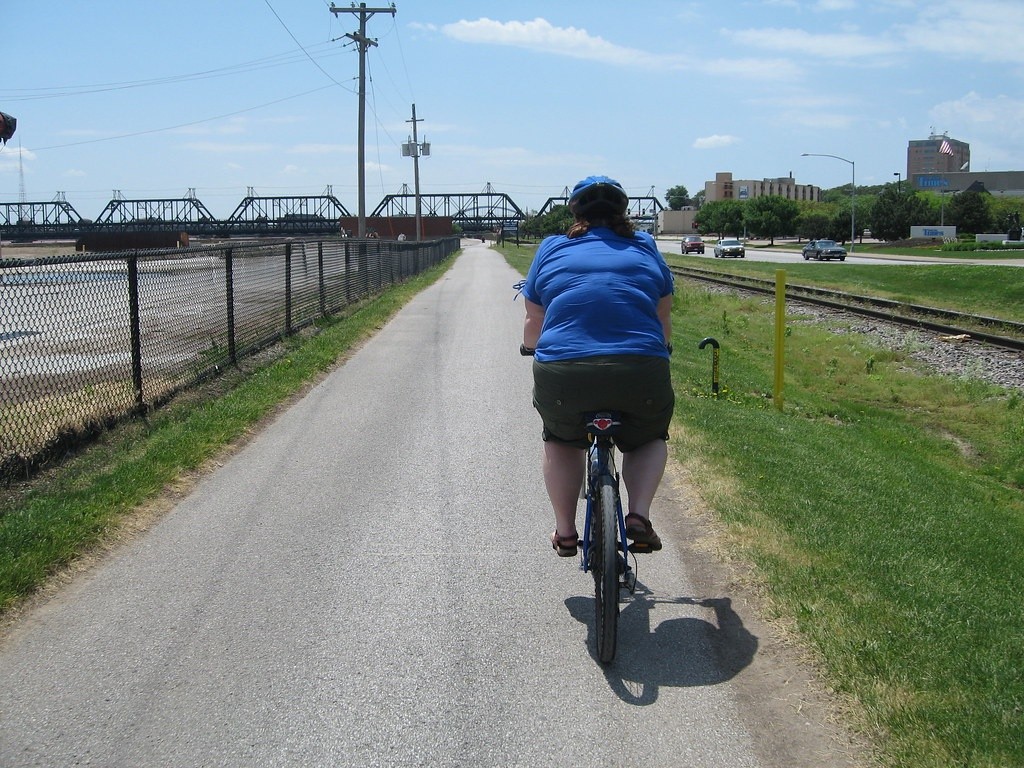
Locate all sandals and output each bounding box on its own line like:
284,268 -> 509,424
550,529 -> 579,557
625,512 -> 662,551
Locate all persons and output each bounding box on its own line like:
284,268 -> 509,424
523,177 -> 674,557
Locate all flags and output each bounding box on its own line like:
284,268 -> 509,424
939,140 -> 954,156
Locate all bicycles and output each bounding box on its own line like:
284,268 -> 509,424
520,344 -> 651,664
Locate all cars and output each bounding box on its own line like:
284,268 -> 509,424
802,240 -> 847,260
714,240 -> 745,259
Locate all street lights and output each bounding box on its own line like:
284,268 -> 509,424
894,172 -> 900,191
712,181 -> 745,245
801,154 -> 855,253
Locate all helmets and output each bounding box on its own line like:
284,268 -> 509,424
568,175 -> 629,217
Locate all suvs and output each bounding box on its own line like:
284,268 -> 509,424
682,236 -> 705,254
